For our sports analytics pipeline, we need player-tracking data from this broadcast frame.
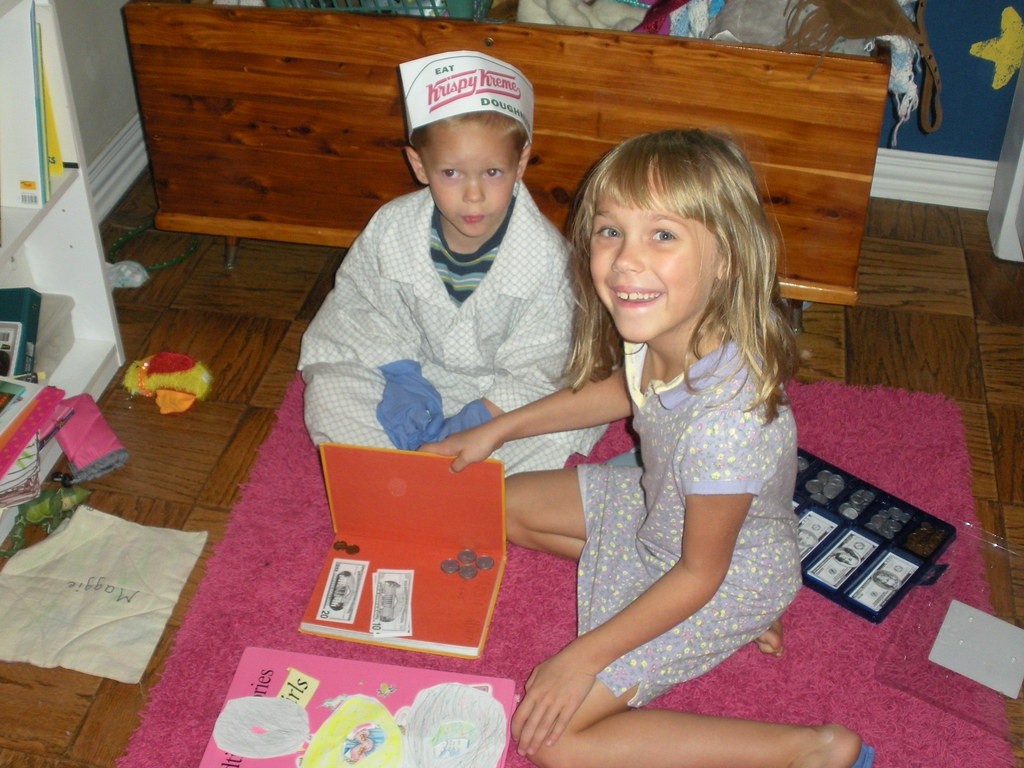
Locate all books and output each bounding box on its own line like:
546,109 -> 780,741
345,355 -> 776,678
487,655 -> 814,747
0,2 -> 63,508
296,442 -> 508,661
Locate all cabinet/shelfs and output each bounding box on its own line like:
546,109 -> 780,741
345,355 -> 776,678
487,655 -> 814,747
0,1 -> 126,551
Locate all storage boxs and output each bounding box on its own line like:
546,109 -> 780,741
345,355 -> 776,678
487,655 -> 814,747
0,287 -> 41,383
33,292 -> 74,385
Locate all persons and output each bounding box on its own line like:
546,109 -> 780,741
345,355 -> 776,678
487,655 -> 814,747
297,50 -> 608,480
416,128 -> 875,768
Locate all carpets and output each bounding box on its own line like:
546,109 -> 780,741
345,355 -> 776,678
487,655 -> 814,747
120,377 -> 1017,768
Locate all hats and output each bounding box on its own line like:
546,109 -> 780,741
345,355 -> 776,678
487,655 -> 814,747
398,49 -> 533,147
